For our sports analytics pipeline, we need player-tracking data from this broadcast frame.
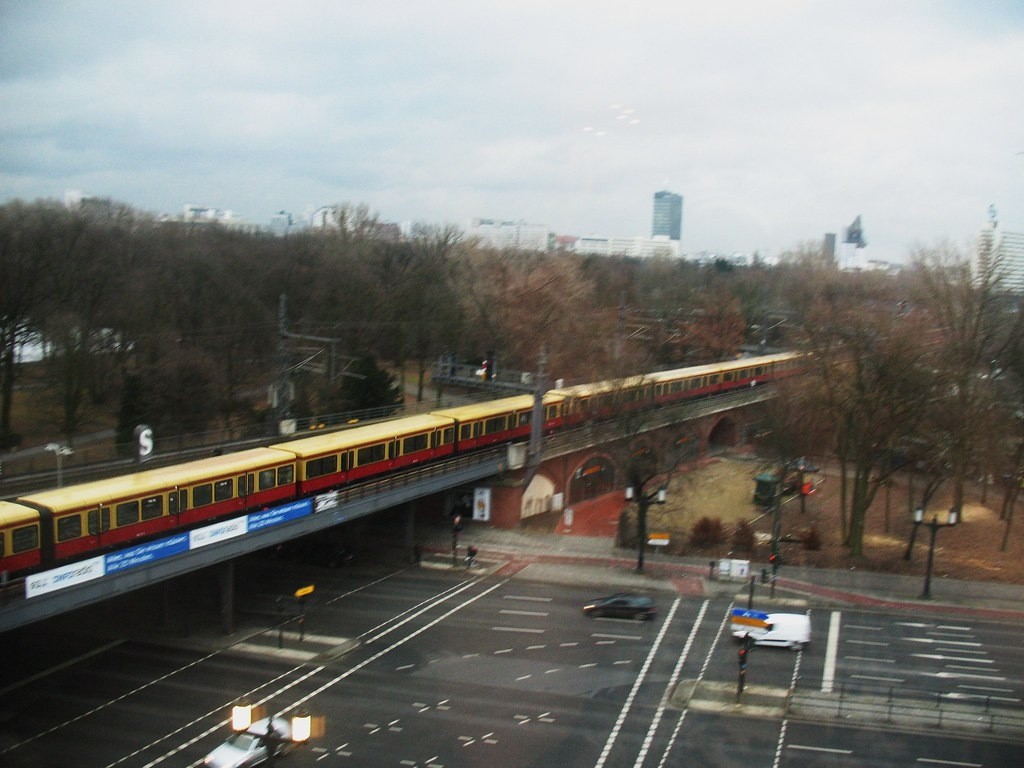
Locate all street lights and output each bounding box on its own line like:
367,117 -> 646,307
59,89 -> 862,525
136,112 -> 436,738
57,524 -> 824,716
625,486 -> 667,576
230,695 -> 311,768
911,505 -> 957,600
44,443 -> 76,490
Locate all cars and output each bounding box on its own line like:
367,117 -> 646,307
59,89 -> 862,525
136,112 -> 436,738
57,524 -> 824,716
582,591 -> 658,621
203,716 -> 297,768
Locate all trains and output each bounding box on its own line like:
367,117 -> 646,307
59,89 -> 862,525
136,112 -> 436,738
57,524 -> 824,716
0,328 -> 947,581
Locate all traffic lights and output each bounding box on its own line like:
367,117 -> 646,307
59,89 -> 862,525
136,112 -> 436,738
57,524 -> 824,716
453,512 -> 462,533
481,353 -> 494,382
770,554 -> 779,562
738,645 -> 746,655
